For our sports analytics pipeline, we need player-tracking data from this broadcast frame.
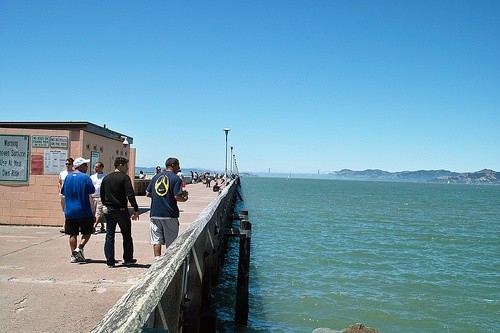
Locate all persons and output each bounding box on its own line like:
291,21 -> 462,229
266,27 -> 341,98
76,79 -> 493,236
189,172 -> 239,192
139,171 -> 144,179
146,158 -> 188,259
61,158 -> 95,263
90,161 -> 107,234
100,157 -> 139,266
58,158 -> 74,233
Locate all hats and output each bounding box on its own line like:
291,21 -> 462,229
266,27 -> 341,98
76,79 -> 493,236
73,158 -> 90,168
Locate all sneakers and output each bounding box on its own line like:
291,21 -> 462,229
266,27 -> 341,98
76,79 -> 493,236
72,248 -> 86,263
71,254 -> 77,263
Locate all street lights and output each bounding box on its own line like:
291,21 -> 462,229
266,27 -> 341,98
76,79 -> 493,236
232,154 -> 239,176
230,145 -> 234,182
222,127 -> 231,186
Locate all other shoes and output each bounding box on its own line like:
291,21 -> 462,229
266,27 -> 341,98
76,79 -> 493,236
60,229 -> 65,232
124,259 -> 137,265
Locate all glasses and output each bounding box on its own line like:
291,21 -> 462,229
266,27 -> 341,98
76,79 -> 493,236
65,163 -> 71,165
